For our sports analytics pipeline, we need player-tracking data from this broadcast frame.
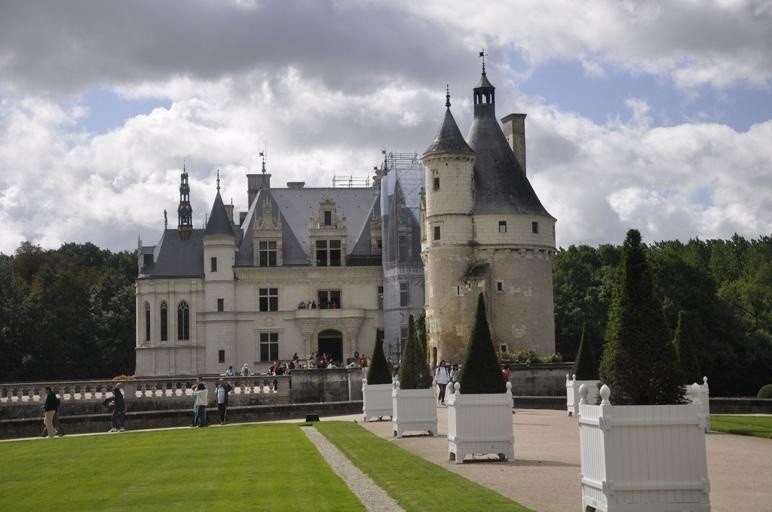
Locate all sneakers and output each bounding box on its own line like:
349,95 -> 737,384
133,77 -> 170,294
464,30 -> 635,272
437,400 -> 446,406
37,430 -> 64,437
109,427 -> 125,432
189,422 -> 229,427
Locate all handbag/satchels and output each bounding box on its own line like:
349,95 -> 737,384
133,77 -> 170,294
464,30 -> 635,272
222,383 -> 232,391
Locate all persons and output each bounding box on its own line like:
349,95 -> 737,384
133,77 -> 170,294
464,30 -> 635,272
449,363 -> 459,395
298,298 -> 340,309
42,385 -> 60,438
434,359 -> 451,406
502,365 -> 517,416
191,348 -> 370,427
111,382 -> 128,431
109,386 -> 125,432
39,391 -> 66,437
